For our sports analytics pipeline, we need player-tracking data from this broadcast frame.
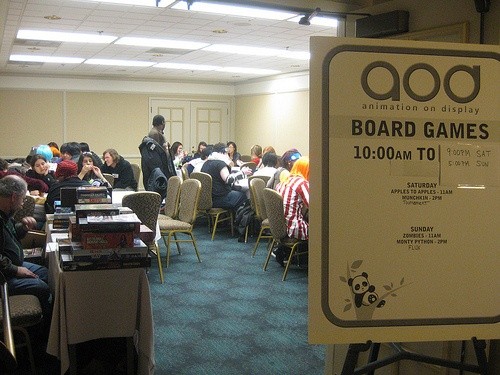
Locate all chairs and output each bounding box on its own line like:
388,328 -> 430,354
248,175 -> 272,222
262,188 -> 308,283
130,163 -> 146,191
157,178 -> 203,269
1,279 -> 43,363
249,178 -> 281,260
122,191 -> 165,284
155,175 -> 183,256
189,172 -> 234,241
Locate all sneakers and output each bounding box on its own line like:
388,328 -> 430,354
272,247 -> 290,263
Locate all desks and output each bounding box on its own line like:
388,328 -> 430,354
44,188 -> 153,374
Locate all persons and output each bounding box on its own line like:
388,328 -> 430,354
100,148 -> 138,192
77,152 -> 113,191
120,234 -> 128,249
139,114 -> 177,212
0,141 -> 82,211
1,174 -> 50,306
273,154 -> 308,266
265,149 -> 300,256
191,141 -> 278,245
80,143 -> 103,170
169,140 -> 186,185
